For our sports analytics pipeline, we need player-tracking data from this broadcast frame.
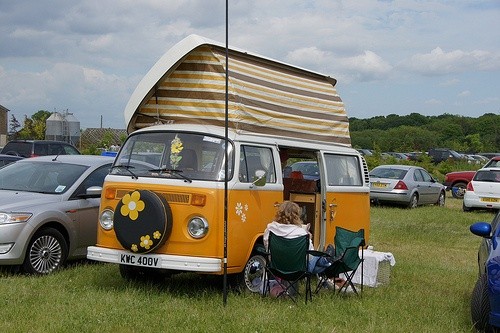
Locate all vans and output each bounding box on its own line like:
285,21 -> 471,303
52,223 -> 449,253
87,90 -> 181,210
89,33 -> 372,286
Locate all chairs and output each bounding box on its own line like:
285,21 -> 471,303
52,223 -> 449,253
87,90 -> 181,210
314,227 -> 364,296
257,233 -> 313,303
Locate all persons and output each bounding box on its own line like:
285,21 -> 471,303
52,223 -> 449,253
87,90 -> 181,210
262,201 -> 315,273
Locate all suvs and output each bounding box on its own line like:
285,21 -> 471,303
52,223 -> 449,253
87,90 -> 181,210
3,141 -> 82,158
442,156 -> 500,199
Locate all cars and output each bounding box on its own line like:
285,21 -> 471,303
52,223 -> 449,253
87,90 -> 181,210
0,154 -> 24,166
470,209 -> 500,333
0,154 -> 160,275
283,162 -> 322,192
459,153 -> 500,164
357,148 -> 428,161
369,165 -> 446,209
428,149 -> 469,162
461,167 -> 500,212
